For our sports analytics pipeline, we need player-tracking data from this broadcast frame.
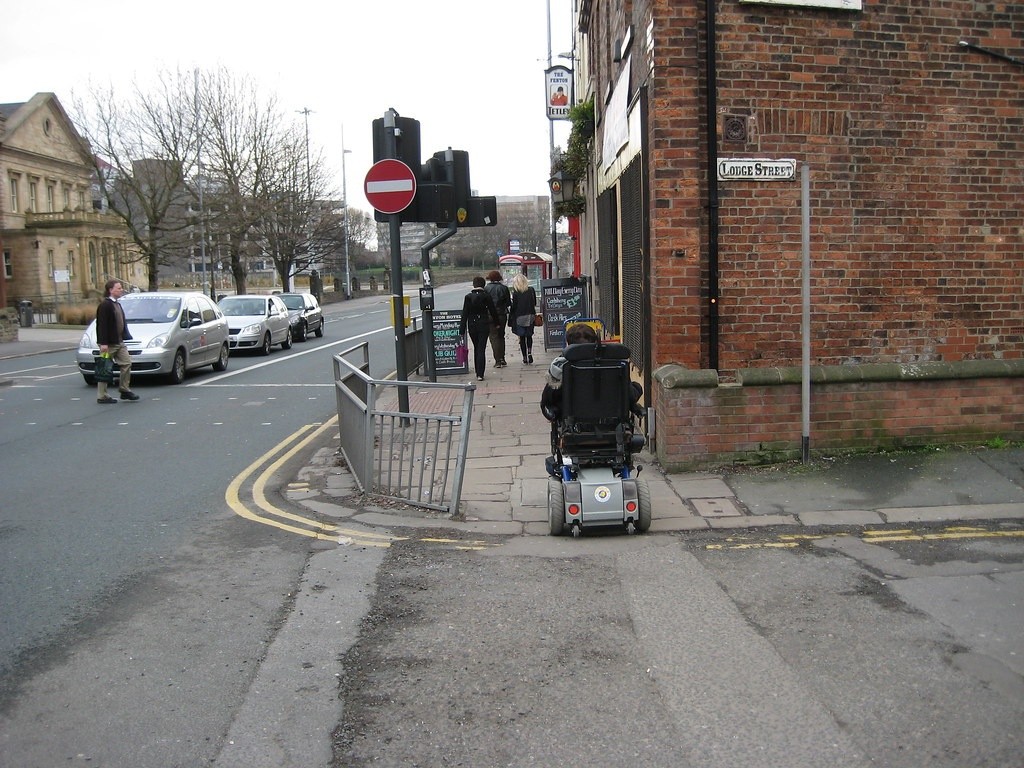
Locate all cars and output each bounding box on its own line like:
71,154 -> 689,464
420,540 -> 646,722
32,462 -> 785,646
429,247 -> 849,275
215,294 -> 293,356
274,293 -> 324,342
76,290 -> 230,387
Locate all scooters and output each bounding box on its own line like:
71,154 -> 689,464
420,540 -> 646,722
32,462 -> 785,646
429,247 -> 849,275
539,327 -> 652,536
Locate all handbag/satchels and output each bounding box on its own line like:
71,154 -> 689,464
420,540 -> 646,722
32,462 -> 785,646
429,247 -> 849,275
456,345 -> 467,367
95,350 -> 114,382
534,315 -> 542,326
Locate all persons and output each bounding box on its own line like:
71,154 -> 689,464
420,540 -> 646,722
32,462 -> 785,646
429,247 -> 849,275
508,273 -> 537,365
542,324 -> 643,413
484,270 -> 512,367
459,276 -> 500,381
96,281 -> 140,403
551,87 -> 567,105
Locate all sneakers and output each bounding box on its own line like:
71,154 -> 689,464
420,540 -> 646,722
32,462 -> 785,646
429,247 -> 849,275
97,397 -> 117,403
121,392 -> 139,400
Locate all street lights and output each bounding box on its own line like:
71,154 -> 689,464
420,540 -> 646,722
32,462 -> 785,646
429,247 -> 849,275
343,149 -> 353,299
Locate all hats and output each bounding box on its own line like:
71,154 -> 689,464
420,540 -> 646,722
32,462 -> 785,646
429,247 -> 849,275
485,271 -> 501,280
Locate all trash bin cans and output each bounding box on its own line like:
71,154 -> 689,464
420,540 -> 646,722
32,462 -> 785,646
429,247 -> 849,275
19,300 -> 33,328
217,294 -> 227,303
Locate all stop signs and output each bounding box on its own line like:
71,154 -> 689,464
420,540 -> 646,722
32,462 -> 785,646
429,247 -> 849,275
362,157 -> 418,215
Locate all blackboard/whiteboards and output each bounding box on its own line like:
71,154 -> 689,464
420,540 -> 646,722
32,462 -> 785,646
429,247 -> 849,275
423,310 -> 469,375
540,277 -> 587,348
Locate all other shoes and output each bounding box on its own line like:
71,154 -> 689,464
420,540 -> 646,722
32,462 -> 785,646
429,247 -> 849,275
522,360 -> 528,365
493,362 -> 501,368
478,376 -> 483,380
501,361 -> 507,367
528,355 -> 533,363
